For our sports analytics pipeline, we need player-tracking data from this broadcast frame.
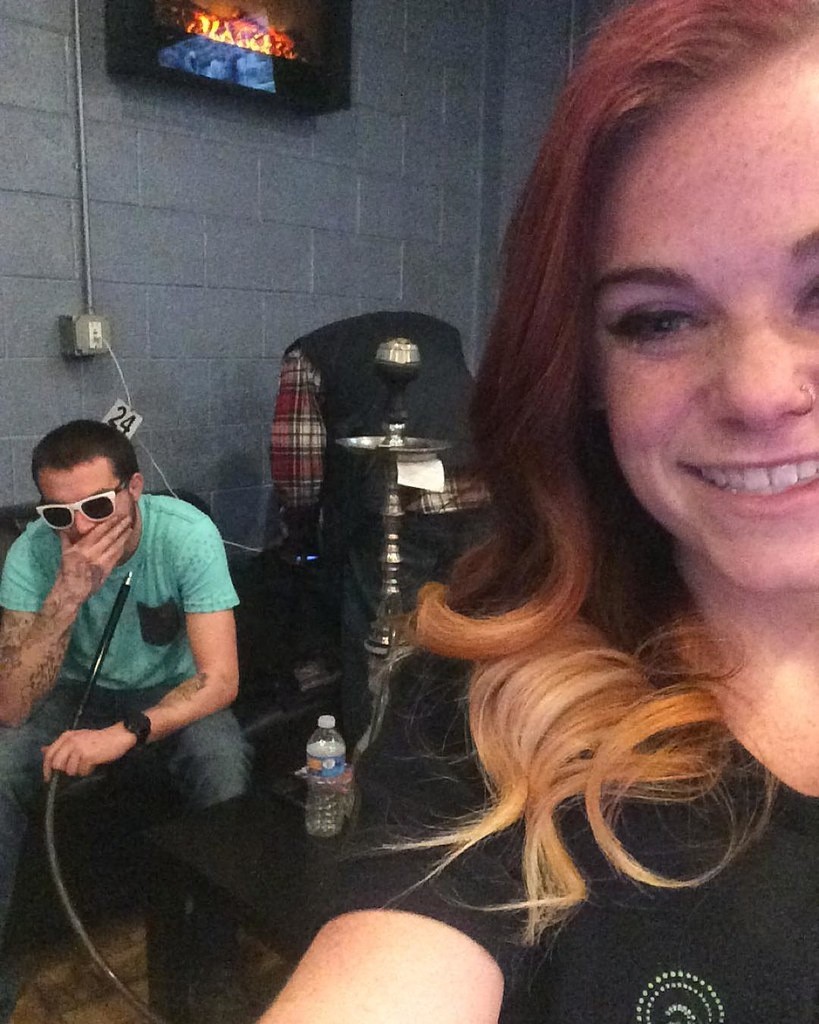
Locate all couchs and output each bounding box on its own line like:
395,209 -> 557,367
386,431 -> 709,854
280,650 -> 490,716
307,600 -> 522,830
0,489 -> 374,945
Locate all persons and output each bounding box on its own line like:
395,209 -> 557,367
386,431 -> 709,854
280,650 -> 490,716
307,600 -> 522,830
253,1 -> 817,1024
1,420 -> 252,1011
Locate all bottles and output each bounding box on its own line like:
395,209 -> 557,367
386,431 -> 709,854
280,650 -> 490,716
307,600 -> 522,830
303,716 -> 347,836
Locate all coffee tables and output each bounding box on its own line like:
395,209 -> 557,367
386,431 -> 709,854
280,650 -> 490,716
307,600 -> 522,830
139,762 -> 355,1024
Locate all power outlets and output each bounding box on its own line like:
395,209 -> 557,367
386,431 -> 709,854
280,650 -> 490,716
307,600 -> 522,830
59,311 -> 111,358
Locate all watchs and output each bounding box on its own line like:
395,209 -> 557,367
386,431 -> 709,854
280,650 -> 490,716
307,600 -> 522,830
123,712 -> 151,747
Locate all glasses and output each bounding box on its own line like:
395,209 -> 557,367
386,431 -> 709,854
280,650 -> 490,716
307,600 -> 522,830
35,478 -> 128,530
